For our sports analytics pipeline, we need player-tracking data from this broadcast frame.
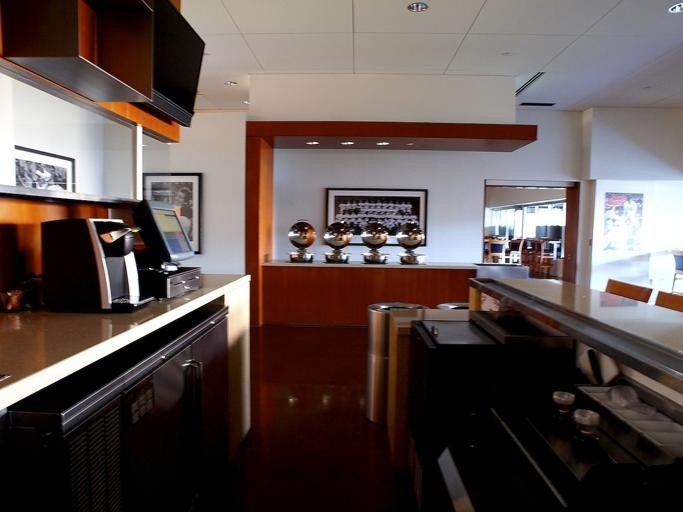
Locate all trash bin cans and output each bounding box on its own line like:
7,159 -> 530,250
366,303 -> 430,426
437,303 -> 469,309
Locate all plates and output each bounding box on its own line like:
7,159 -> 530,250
576,385 -> 682,458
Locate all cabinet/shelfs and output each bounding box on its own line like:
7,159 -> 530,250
0,0 -> 181,144
261,258 -> 478,327
252,326 -> 372,511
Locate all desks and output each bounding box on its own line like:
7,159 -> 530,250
469,273 -> 683,382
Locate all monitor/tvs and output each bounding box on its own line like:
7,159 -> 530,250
148,0 -> 205,128
133,200 -> 194,271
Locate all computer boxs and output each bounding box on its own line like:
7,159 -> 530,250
138,267 -> 201,301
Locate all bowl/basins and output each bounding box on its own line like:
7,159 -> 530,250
552,391 -> 575,405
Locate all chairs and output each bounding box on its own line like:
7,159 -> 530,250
605,277 -> 650,303
484,234 -> 564,278
655,289 -> 678,310
671,249 -> 682,291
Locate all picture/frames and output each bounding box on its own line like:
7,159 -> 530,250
325,187 -> 428,246
15,145 -> 75,192
142,171 -> 202,255
